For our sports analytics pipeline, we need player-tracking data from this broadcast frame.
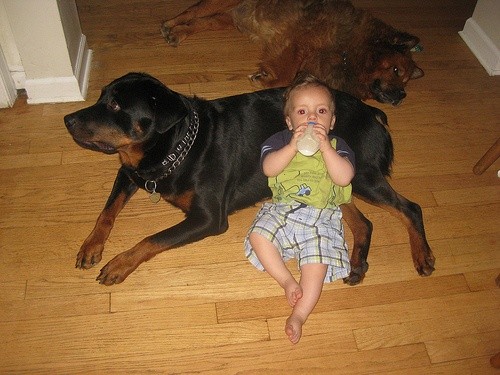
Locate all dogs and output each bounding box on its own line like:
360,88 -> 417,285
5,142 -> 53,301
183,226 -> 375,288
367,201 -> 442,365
160,0 -> 425,107
63,71 -> 436,287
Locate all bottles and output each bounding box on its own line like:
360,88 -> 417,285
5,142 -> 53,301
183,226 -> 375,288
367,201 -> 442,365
296,122 -> 320,157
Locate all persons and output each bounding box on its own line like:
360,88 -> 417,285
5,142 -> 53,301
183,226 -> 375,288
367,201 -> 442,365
243,75 -> 357,345
